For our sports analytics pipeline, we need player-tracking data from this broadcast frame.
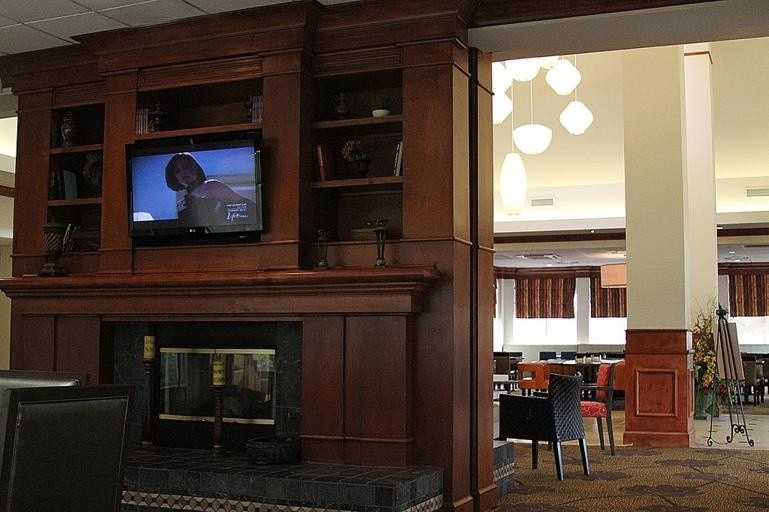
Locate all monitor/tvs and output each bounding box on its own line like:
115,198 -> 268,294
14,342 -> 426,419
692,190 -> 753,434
60,222 -> 84,251
56,167 -> 79,200
345,226 -> 386,241
392,143 -> 400,176
316,142 -> 338,182
395,140 -> 404,176
49,170 -> 59,200
312,144 -> 321,180
134,105 -> 166,135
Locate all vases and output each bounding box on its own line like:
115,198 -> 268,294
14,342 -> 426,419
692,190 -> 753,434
692,294 -> 736,418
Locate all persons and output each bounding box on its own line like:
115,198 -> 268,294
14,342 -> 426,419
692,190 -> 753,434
162,150 -> 254,226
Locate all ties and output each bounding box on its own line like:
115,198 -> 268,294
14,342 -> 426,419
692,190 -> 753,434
125,128 -> 263,236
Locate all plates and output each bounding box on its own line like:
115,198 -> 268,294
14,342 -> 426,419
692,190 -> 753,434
1,0 -> 471,277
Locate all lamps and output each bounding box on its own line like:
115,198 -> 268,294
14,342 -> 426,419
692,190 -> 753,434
499,362 -> 616,481
741,354 -> 765,404
495,352 -> 624,401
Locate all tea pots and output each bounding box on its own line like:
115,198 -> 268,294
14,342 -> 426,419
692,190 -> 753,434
472,35 -> 767,511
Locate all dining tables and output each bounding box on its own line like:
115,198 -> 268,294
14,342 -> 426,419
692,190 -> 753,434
367,94 -> 393,117
